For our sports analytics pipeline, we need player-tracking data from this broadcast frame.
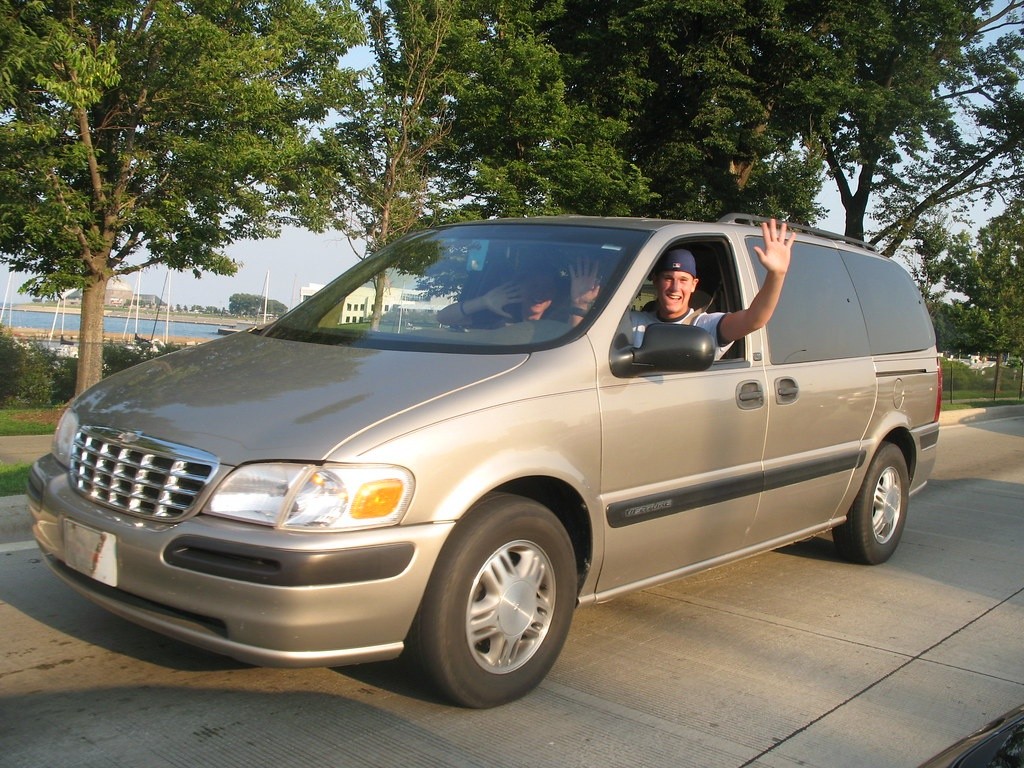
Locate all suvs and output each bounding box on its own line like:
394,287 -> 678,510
23,212 -> 943,709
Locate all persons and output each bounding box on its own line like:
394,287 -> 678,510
568,219 -> 796,361
436,261 -> 567,330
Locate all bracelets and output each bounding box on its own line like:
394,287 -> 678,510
569,306 -> 585,317
461,300 -> 469,317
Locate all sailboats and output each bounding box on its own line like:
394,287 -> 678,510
120,269 -> 150,352
135,270 -> 171,356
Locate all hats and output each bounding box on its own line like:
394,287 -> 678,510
654,249 -> 696,279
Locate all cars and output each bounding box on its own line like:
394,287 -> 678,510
447,325 -> 471,332
406,323 -> 413,329
987,352 -> 1003,361
